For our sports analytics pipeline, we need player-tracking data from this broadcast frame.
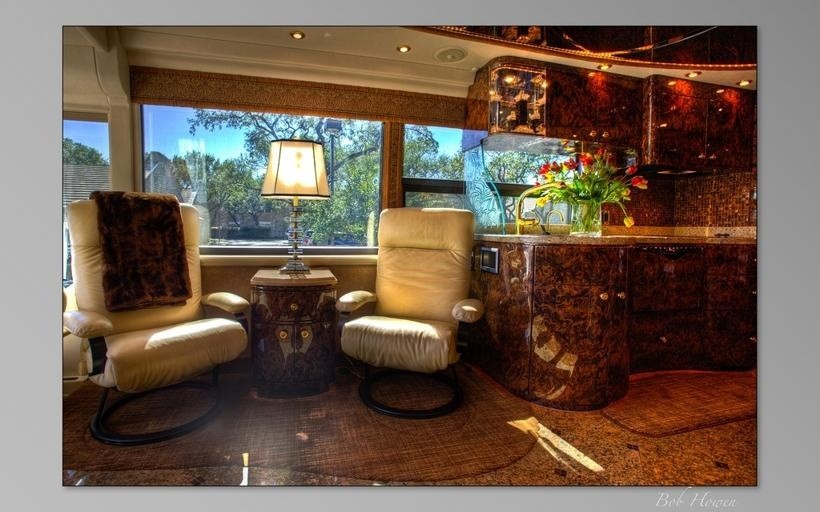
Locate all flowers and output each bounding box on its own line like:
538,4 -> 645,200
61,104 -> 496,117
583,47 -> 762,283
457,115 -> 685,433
533,147 -> 648,228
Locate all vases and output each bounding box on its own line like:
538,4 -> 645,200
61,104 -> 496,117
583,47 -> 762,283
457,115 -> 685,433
569,200 -> 602,237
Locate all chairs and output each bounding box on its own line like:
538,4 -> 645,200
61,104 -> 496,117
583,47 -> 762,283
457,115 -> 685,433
63,189 -> 250,448
334,206 -> 484,420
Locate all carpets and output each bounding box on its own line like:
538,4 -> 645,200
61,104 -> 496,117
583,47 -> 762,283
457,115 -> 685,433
62,366 -> 539,482
600,368 -> 757,439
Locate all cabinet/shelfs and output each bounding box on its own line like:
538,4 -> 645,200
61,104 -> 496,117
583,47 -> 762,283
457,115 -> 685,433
249,267 -> 338,400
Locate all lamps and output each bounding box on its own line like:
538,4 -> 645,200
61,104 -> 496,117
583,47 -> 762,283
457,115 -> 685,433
260,138 -> 330,274
